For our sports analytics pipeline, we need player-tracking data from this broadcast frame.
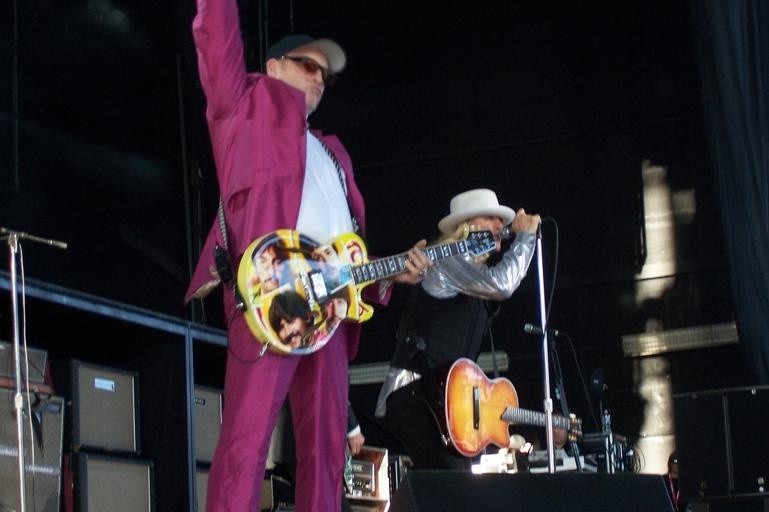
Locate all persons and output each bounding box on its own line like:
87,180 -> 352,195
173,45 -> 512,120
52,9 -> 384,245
266,288 -> 314,356
296,233 -> 341,281
372,188 -> 542,470
247,235 -> 295,291
285,397 -> 366,511
321,278 -> 353,336
178,1 -> 437,511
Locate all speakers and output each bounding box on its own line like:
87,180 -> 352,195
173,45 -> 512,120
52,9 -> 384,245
669,392 -> 729,497
726,387 -> 769,496
387,470 -> 677,512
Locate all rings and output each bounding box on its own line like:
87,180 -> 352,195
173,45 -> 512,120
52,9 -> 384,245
419,270 -> 425,277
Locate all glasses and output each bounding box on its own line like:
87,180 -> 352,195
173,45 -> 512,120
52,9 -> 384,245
285,55 -> 330,82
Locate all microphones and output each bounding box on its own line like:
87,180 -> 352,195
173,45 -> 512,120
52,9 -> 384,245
502,219 -> 543,237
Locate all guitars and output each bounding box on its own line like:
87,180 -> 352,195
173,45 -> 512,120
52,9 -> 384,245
438,357 -> 582,457
234,222 -> 496,356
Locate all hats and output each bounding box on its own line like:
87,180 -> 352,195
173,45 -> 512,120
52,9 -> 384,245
438,187 -> 517,235
262,34 -> 346,78
